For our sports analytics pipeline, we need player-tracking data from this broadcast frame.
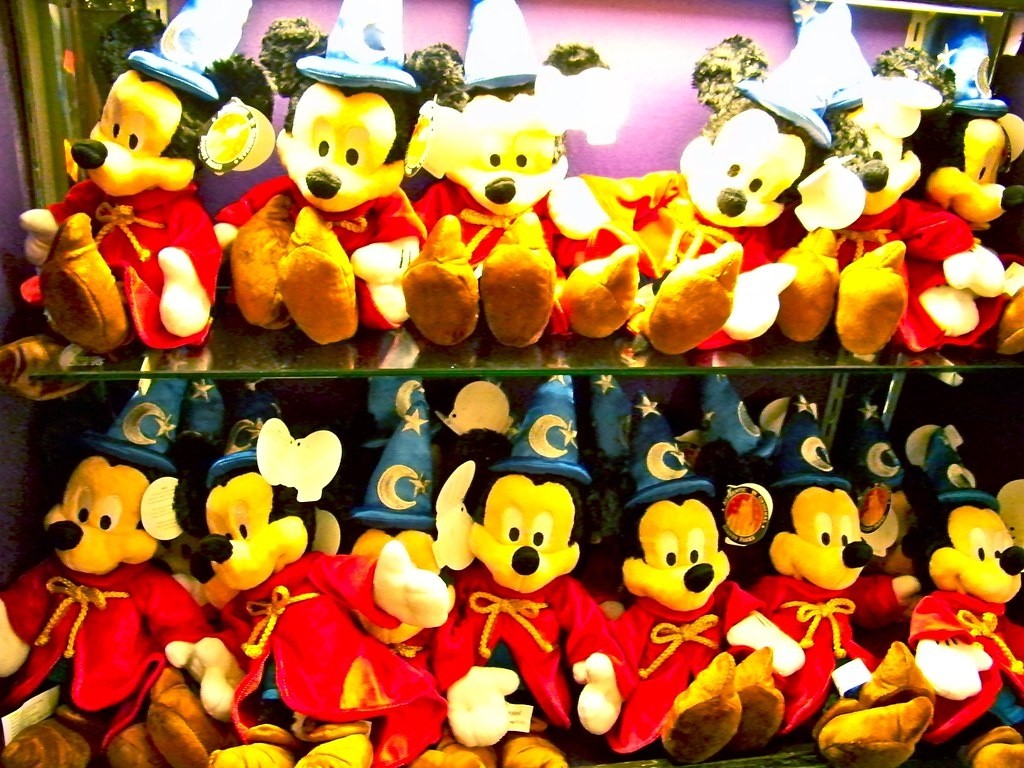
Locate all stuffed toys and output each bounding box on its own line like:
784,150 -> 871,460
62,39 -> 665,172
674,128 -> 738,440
405,0 -> 569,346
0,352 -> 1024,768
546,0 -> 871,355
213,0 -> 425,346
785,0 -> 1024,355
18,0 -> 275,352
